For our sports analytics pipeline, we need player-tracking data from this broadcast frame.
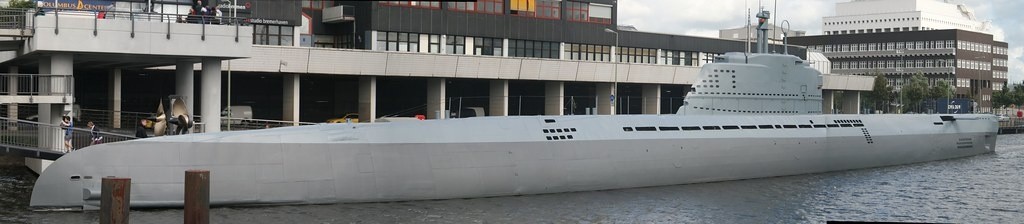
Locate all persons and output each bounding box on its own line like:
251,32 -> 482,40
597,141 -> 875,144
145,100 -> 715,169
136,119 -> 149,138
176,0 -> 223,24
86,121 -> 104,145
60,116 -> 74,154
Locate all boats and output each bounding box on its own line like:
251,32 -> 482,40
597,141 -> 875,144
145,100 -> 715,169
29,0 -> 998,212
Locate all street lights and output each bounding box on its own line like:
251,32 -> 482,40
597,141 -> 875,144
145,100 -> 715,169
604,28 -> 618,116
